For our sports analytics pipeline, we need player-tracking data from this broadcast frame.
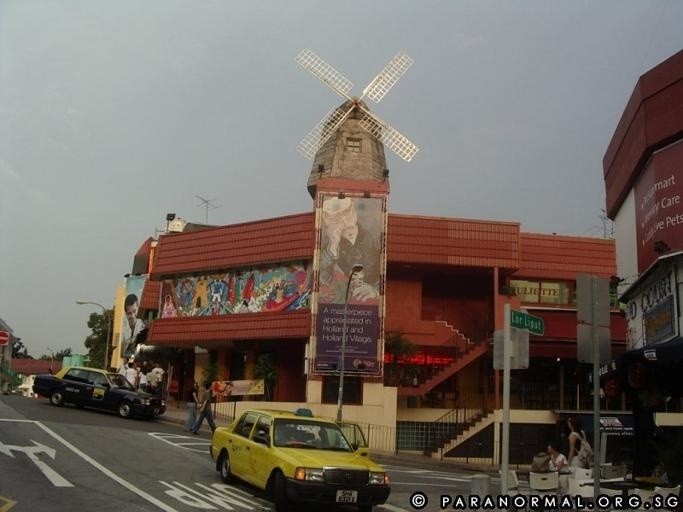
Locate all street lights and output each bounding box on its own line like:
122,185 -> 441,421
76,301 -> 110,371
336,263 -> 364,422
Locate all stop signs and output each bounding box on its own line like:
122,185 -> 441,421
0,331 -> 10,346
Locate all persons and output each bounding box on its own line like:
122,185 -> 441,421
119,356 -> 166,397
318,195 -> 381,302
182,380 -> 216,436
121,293 -> 147,352
527,416 -> 587,475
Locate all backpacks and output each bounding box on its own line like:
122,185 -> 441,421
572,430 -> 592,465
531,453 -> 549,473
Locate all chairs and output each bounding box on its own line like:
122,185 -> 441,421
498,462 -> 682,508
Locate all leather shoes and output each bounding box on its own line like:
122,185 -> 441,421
184,429 -> 200,435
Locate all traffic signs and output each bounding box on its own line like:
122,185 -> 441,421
511,308 -> 549,340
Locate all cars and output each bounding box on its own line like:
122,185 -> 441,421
209,408 -> 391,512
32,367 -> 166,419
